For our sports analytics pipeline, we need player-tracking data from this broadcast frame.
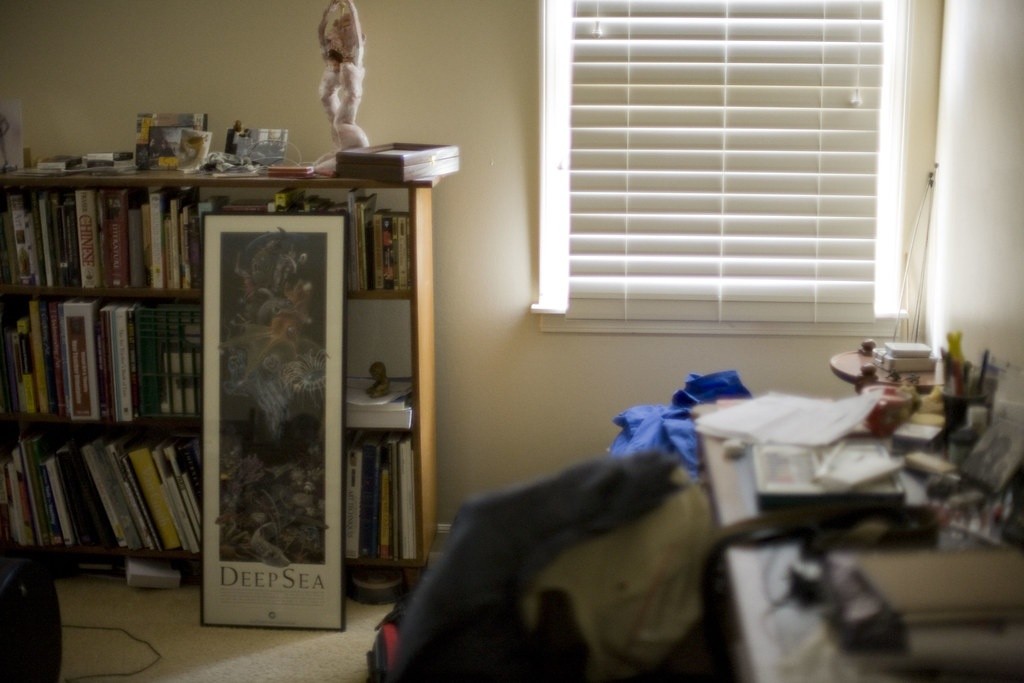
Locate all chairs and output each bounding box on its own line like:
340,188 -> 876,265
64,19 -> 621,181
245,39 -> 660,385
387,451 -> 729,683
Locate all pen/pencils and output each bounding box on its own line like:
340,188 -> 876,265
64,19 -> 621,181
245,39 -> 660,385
940,331 -> 990,397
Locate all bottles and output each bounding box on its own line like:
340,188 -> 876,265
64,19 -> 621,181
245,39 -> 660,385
963,406 -> 988,436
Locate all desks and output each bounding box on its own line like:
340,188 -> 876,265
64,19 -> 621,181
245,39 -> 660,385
828,339 -> 945,395
687,403 -> 1024,683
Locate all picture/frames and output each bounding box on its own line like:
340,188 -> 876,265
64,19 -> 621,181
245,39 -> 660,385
198,209 -> 346,632
134,112 -> 208,171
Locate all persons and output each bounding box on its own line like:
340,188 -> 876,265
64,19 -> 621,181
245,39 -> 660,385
316,1 -> 370,149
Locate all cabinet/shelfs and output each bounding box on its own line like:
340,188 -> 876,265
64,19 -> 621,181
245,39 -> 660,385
0,166 -> 438,590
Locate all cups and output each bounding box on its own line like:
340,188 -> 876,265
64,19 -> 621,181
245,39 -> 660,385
861,386 -> 916,437
940,387 -> 994,467
947,432 -> 977,466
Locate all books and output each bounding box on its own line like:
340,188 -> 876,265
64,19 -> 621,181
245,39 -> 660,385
0,191 -> 416,559
125,556 -> 181,589
79,563 -> 112,570
